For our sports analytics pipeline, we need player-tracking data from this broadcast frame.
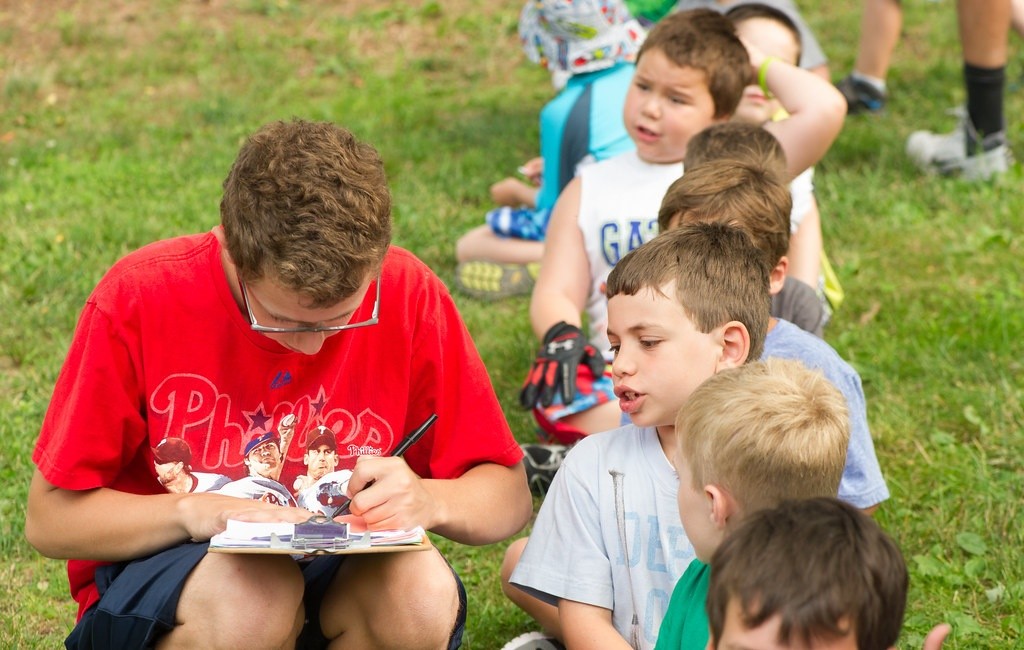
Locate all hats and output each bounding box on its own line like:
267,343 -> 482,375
518,0 -> 647,92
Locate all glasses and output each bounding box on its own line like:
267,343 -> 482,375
236,272 -> 380,333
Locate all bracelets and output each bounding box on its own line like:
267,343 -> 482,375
759,57 -> 781,100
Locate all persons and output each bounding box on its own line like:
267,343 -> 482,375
652,357 -> 850,650
704,497 -> 909,650
724,3 -> 823,295
657,159 -> 892,518
683,122 -> 825,338
153,422 -> 354,518
836,0 -> 1024,115
669,0 -> 830,81
23,117 -> 534,650
454,0 -> 648,301
904,0 -> 1012,180
509,219 -> 775,650
520,8 -> 846,498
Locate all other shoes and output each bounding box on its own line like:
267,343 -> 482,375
454,259 -> 541,302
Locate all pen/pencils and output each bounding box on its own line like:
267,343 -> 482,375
329,413 -> 439,520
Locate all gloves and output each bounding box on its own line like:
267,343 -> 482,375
519,321 -> 605,409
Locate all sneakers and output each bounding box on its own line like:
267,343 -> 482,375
521,441 -> 582,496
905,129 -> 1008,183
833,76 -> 890,114
